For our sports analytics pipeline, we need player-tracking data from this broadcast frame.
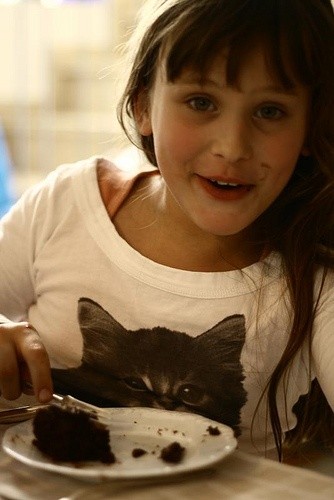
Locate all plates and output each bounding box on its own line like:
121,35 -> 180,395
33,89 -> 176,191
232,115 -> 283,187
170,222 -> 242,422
2,407 -> 238,477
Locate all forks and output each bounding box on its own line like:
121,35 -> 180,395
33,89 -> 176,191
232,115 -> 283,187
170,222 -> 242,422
21,381 -> 111,430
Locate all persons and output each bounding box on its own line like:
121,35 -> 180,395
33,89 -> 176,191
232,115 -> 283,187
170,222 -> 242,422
0,0 -> 334,464
